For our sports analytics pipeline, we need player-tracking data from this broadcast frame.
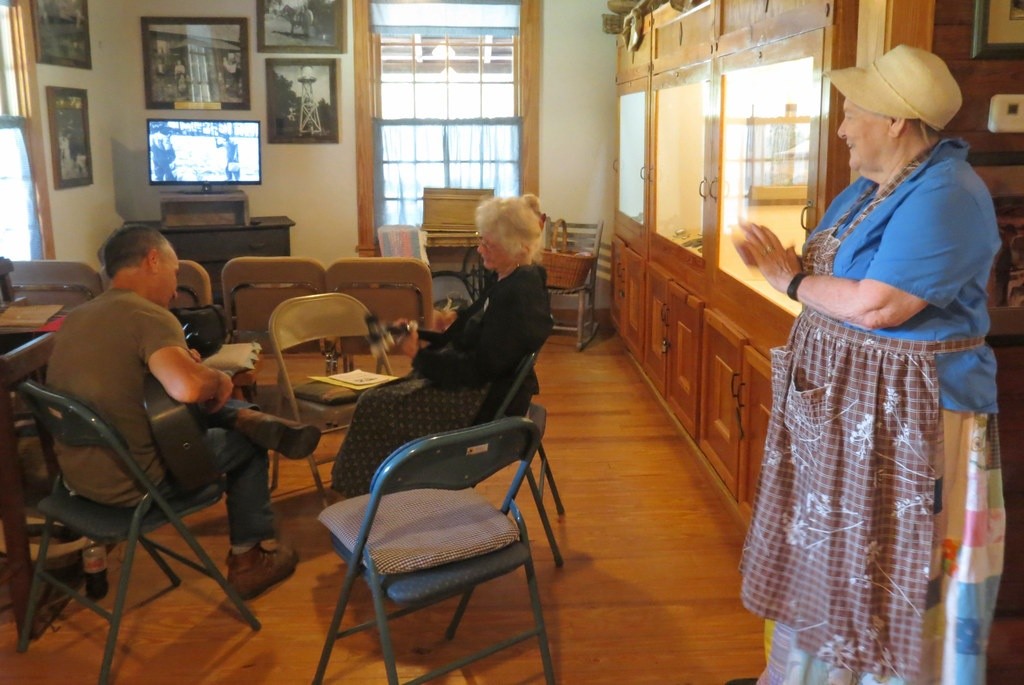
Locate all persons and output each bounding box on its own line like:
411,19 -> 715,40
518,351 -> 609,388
43,226 -> 323,602
331,198 -> 552,501
731,42 -> 1005,685
151,126 -> 240,182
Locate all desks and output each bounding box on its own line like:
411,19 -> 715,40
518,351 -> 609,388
124,214 -> 298,262
419,230 -> 491,303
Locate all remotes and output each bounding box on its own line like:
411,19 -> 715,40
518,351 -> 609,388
250,220 -> 261,225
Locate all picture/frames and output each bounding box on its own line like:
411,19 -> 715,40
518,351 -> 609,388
971,0 -> 1024,61
32,0 -> 92,70
139,16 -> 251,111
266,57 -> 343,146
44,85 -> 97,189
968,150 -> 1024,347
257,0 -> 348,54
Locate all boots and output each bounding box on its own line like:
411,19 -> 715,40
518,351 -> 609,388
235,407 -> 322,460
225,539 -> 298,600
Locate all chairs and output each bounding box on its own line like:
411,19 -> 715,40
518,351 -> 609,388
97,258 -> 213,306
326,257 -> 432,372
16,373 -> 269,685
220,257 -> 324,373
306,415 -> 555,685
266,293 -> 399,505
533,217 -> 605,352
5,257 -> 100,304
0,324 -> 61,628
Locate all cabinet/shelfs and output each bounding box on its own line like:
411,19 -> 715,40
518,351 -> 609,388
603,0 -> 860,526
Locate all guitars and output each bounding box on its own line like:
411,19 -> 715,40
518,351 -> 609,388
363,307 -> 464,348
143,373 -> 224,507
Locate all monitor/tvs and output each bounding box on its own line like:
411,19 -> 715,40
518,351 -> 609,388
147,118 -> 262,194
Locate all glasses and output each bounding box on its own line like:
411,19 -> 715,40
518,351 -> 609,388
475,230 -> 505,254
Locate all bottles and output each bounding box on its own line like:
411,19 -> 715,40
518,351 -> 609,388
82,539 -> 108,600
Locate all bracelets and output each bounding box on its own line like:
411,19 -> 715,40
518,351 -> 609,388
787,272 -> 807,302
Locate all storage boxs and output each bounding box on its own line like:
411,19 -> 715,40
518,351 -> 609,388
422,187 -> 494,232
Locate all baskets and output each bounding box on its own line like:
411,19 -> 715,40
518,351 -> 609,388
539,220 -> 595,289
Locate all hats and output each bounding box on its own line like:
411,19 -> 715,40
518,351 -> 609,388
828,45 -> 962,130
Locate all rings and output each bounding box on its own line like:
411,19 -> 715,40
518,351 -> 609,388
765,246 -> 773,252
761,249 -> 767,255
